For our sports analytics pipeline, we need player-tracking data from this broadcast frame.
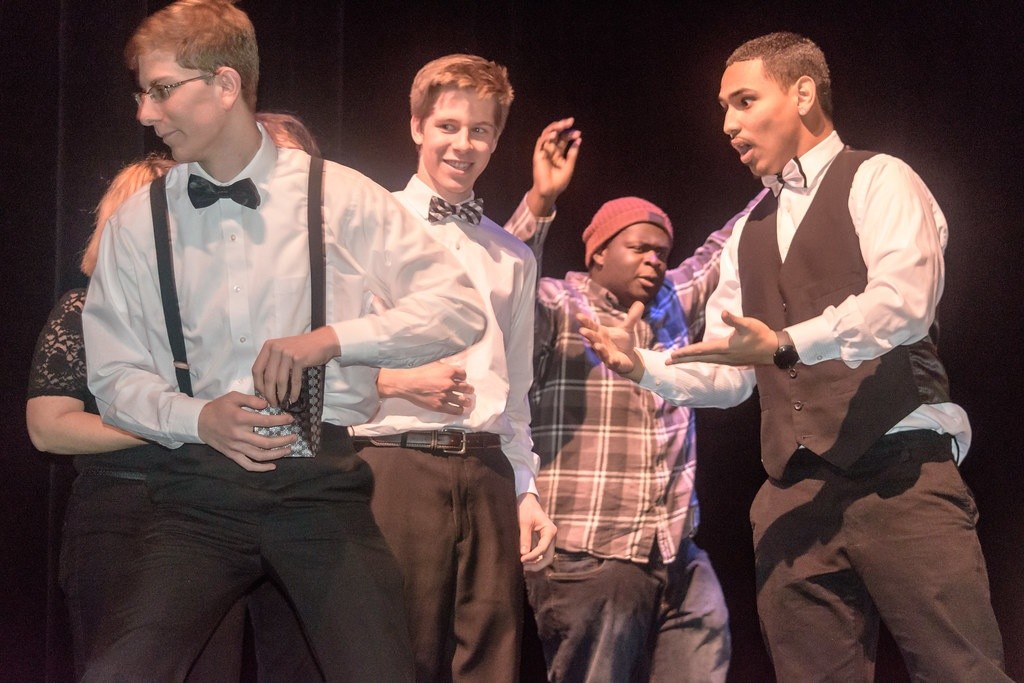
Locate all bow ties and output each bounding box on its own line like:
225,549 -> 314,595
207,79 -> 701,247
428,197 -> 485,226
760,156 -> 808,200
187,174 -> 260,209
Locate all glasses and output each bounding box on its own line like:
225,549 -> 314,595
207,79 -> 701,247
133,73 -> 214,107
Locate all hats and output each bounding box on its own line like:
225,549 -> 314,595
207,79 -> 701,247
582,196 -> 673,269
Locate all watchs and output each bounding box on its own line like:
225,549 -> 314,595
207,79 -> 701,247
772,330 -> 801,371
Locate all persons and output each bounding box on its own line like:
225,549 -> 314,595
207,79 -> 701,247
25,111 -> 325,683
574,30 -> 1024,683
79,0 -> 489,683
499,115 -> 771,683
349,50 -> 559,683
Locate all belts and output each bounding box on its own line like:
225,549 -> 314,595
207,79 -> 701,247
351,433 -> 501,454
